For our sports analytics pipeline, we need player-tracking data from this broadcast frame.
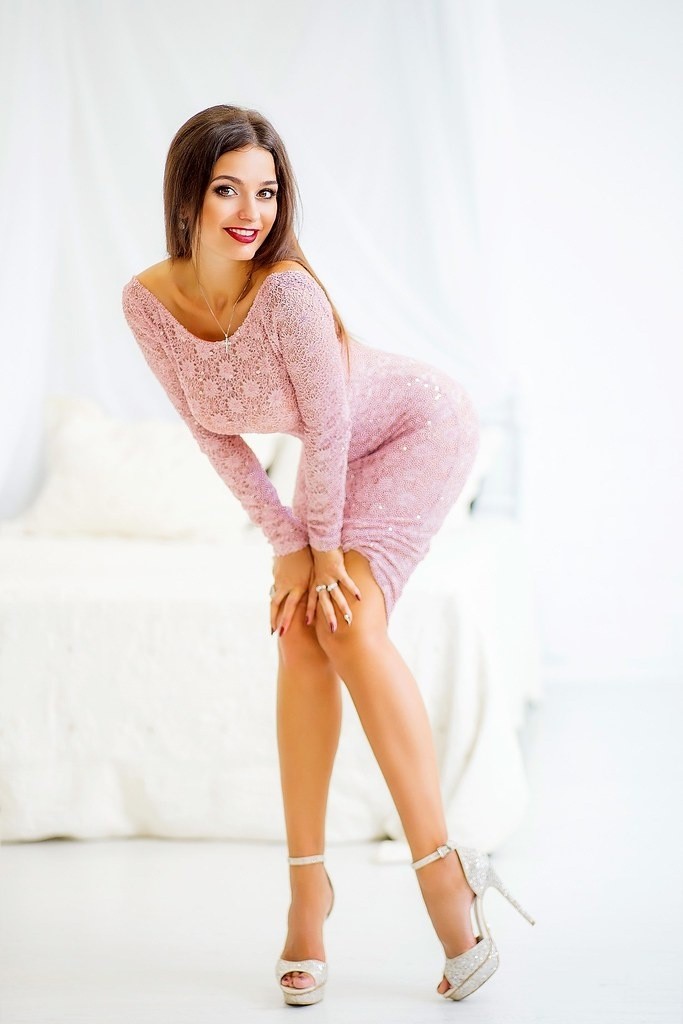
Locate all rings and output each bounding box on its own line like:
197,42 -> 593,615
315,583 -> 340,592
269,585 -> 276,597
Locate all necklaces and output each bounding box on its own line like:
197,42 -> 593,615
189,258 -> 255,354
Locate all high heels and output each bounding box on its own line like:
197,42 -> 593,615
412,839 -> 537,1000
275,854 -> 337,1008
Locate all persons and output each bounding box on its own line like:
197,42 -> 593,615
122,104 -> 537,1008
